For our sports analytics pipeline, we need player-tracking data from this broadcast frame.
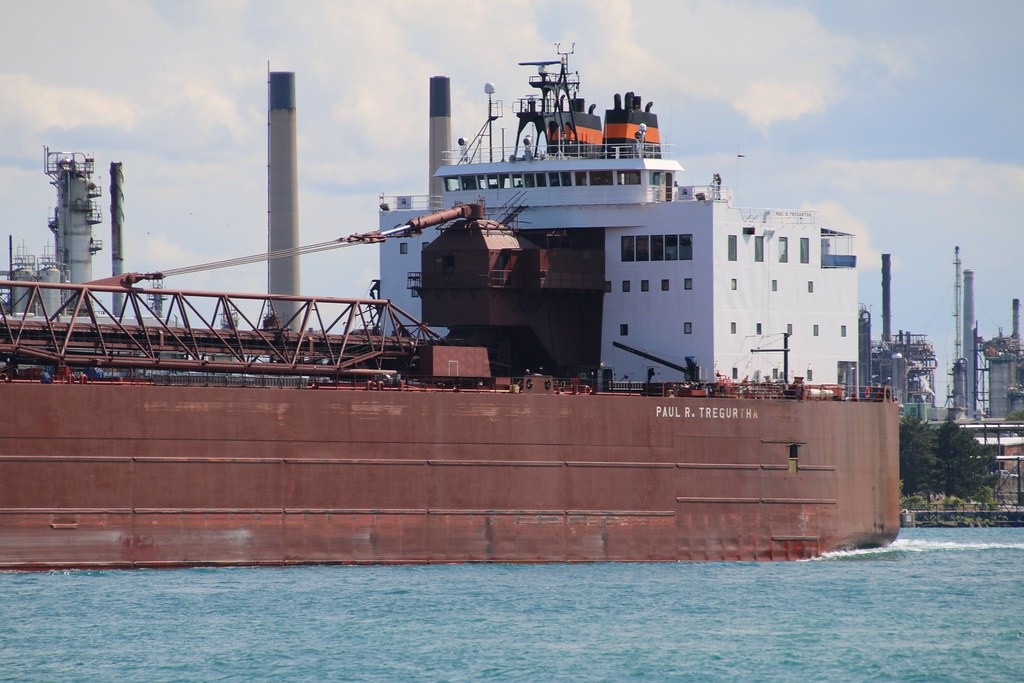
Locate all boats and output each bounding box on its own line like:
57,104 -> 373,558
0,36 -> 902,571
